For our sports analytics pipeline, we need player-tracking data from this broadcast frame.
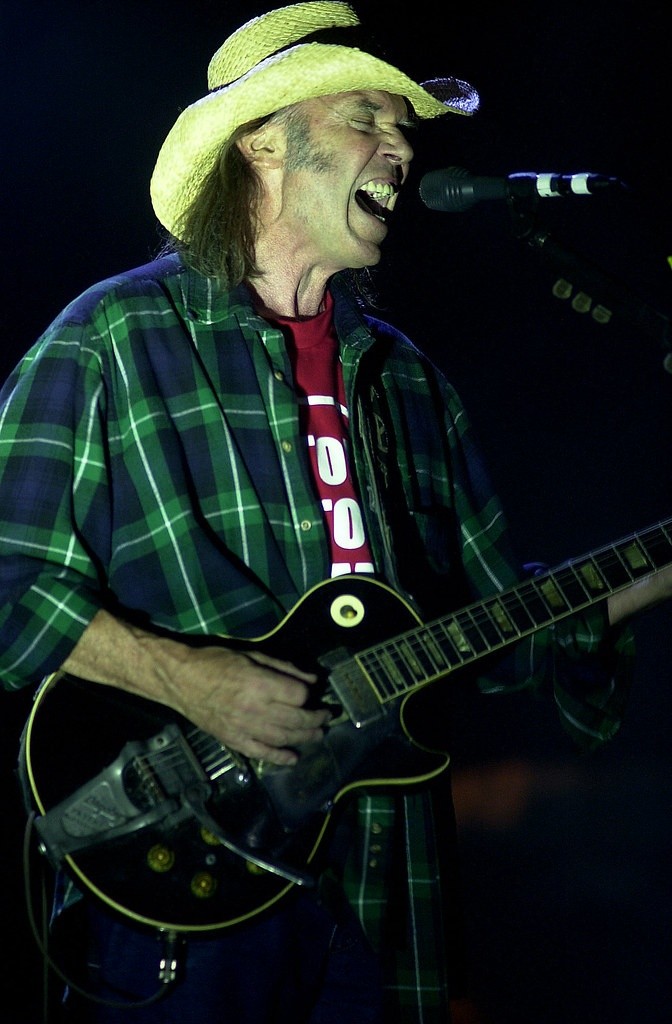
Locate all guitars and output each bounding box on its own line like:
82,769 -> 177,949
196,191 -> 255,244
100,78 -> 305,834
19,515 -> 672,936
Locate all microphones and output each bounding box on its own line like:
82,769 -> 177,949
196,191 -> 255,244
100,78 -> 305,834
420,167 -> 623,210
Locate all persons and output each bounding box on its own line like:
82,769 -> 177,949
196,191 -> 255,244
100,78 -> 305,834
0,0 -> 672,1024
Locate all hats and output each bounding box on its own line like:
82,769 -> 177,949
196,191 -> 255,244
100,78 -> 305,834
149,0 -> 481,247
